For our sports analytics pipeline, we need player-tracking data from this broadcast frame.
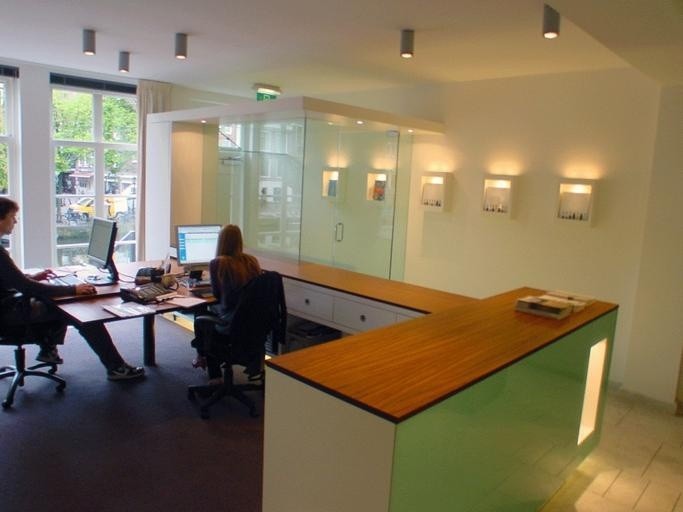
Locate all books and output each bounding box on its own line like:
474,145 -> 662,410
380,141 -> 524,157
514,285 -> 598,321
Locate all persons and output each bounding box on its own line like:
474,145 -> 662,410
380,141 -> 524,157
1,196 -> 146,381
190,223 -> 260,384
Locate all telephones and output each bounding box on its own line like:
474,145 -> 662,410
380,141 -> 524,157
118,282 -> 171,304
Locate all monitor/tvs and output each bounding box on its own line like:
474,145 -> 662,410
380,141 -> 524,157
83,218 -> 119,285
175,224 -> 223,287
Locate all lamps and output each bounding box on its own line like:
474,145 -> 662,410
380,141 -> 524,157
398,31 -> 417,59
116,52 -> 132,74
175,35 -> 190,61
80,29 -> 96,54
538,8 -> 564,41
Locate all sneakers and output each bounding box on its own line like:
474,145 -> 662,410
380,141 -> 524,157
107,363 -> 145,382
34,349 -> 64,365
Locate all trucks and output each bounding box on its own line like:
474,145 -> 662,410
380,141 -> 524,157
68,195 -> 129,221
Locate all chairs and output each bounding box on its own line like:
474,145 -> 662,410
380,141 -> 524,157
188,272 -> 282,418
0,275 -> 73,406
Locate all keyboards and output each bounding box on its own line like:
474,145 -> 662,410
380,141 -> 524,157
48,273 -> 86,287
193,289 -> 214,297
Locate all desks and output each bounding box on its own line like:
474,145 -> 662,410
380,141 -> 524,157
260,285 -> 634,511
255,252 -> 480,334
23,255 -> 214,367
